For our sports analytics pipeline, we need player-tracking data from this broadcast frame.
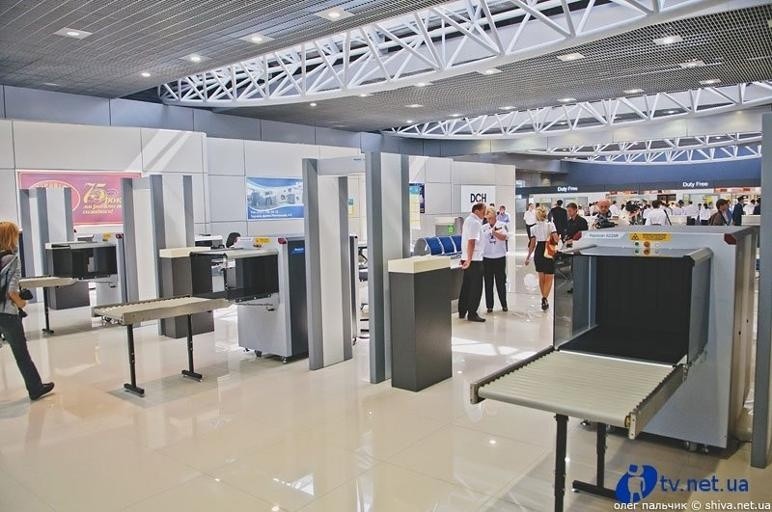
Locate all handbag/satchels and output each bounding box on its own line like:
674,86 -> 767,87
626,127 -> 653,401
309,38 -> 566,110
544,235 -> 563,259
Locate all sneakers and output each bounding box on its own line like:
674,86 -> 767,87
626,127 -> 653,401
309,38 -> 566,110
542,297 -> 549,310
30,383 -> 54,400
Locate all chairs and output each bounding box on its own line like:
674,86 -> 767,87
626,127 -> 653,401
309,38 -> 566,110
412,235 -> 463,258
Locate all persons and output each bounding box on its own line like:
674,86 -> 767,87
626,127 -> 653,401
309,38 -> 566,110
0,221 -> 55,401
457,192 -> 763,324
225,232 -> 241,248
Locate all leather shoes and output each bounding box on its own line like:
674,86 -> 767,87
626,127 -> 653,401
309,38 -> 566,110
468,313 -> 485,322
